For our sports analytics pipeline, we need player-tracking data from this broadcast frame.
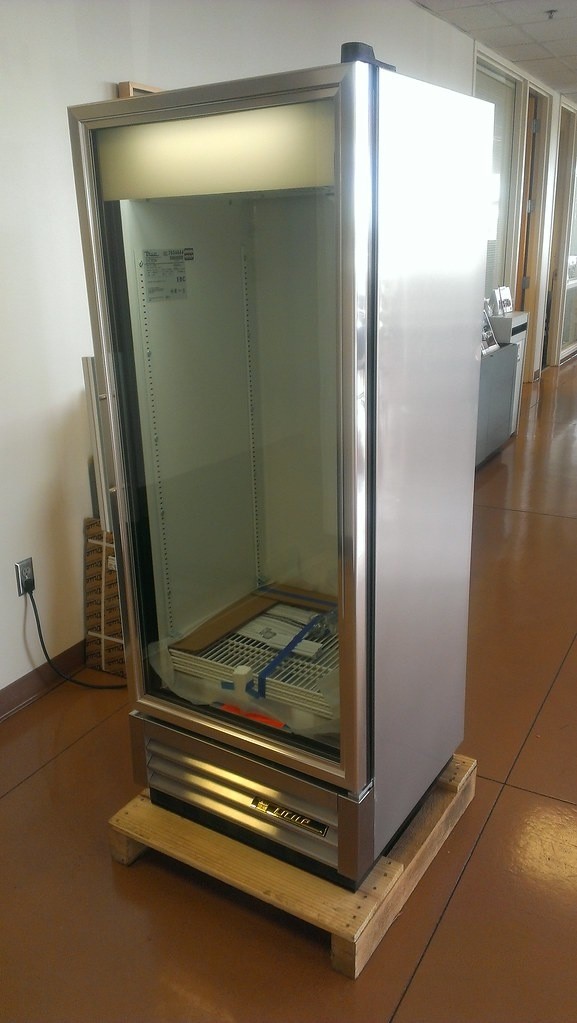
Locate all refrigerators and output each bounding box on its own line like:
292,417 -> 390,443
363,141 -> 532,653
67,44 -> 495,881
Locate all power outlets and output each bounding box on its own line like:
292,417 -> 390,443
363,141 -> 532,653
15,557 -> 35,596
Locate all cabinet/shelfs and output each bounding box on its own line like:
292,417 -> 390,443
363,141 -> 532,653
490,310 -> 530,436
